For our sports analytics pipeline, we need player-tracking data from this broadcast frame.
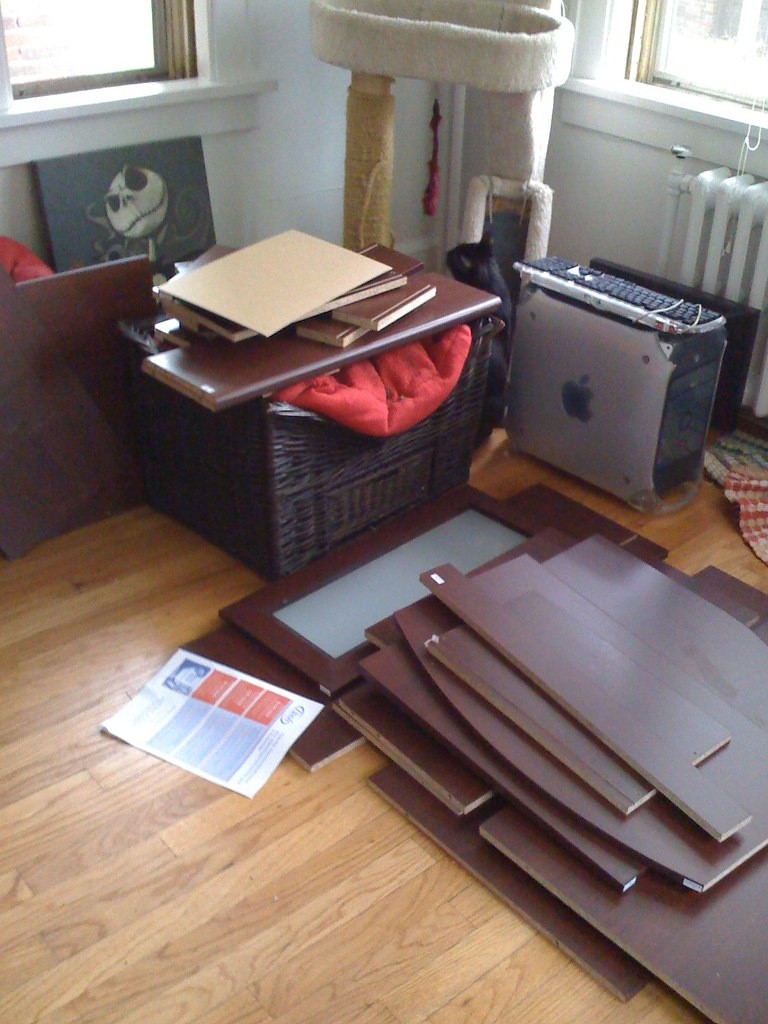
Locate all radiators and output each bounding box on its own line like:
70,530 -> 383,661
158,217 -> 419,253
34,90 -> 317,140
658,167 -> 768,423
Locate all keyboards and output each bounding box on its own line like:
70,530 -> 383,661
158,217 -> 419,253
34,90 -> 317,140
512,257 -> 725,335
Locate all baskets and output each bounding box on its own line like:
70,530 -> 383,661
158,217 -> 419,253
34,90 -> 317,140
118,312 -> 505,581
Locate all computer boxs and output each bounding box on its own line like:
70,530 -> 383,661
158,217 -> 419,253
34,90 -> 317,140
504,285 -> 728,508
